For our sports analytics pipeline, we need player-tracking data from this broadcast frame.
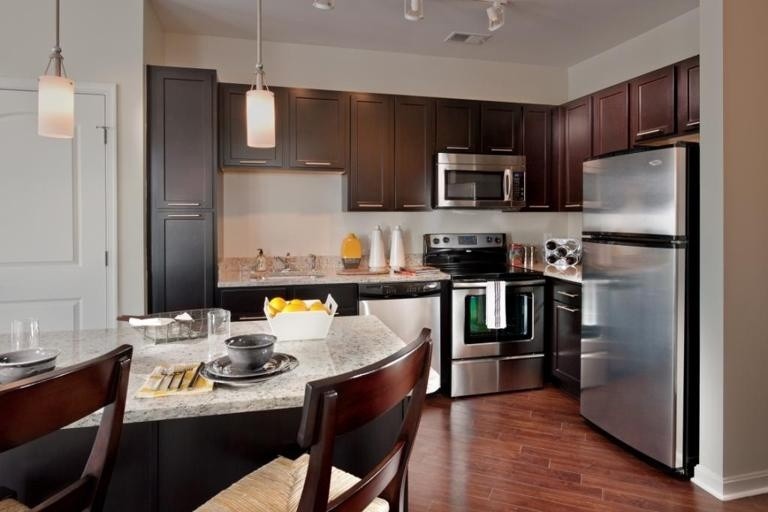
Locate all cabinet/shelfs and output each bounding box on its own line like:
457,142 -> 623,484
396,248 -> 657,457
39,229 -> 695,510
340,93 -> 433,213
546,278 -> 582,394
523,102 -> 554,213
220,82 -> 350,175
148,209 -> 218,314
630,56 -> 700,155
146,63 -> 218,209
433,98 -> 523,155
219,284 -> 359,321
554,94 -> 594,211
594,80 -> 630,159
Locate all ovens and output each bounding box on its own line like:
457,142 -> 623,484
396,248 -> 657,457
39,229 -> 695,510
441,282 -> 547,399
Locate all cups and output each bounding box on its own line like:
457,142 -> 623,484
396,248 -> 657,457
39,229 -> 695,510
205,311 -> 231,360
9,319 -> 41,352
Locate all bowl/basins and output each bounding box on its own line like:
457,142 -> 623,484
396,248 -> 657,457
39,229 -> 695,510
224,332 -> 277,369
0,347 -> 62,385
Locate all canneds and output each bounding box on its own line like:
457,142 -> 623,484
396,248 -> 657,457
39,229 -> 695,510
508,243 -> 523,266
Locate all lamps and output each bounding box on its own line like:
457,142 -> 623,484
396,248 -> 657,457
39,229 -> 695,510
35,1 -> 77,140
243,1 -> 278,149
313,1 -> 509,33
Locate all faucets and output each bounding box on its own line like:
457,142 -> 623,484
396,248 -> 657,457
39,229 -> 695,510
275,252 -> 293,272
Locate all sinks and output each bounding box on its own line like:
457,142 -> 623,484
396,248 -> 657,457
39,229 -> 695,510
250,274 -> 321,285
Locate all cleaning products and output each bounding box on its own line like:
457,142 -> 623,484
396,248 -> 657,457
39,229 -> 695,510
256,248 -> 266,270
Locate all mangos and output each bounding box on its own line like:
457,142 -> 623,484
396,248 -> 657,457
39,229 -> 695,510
267,297 -> 331,317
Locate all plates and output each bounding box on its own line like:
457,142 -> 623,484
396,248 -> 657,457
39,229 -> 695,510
198,352 -> 300,388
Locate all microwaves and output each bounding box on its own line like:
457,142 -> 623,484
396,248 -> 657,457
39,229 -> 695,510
433,152 -> 528,212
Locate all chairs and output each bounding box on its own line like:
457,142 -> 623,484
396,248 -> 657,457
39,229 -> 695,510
190,327 -> 434,511
1,343 -> 135,510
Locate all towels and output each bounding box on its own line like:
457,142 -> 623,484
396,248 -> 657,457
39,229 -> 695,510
484,278 -> 508,331
132,363 -> 216,400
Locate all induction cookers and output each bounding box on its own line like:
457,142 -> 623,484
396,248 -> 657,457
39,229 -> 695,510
426,265 -> 543,281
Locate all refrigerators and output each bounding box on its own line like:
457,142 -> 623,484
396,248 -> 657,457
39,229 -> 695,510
581,139 -> 700,478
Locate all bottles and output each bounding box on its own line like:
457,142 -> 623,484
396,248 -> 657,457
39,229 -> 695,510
368,225 -> 407,270
339,231 -> 363,271
509,242 -> 535,267
